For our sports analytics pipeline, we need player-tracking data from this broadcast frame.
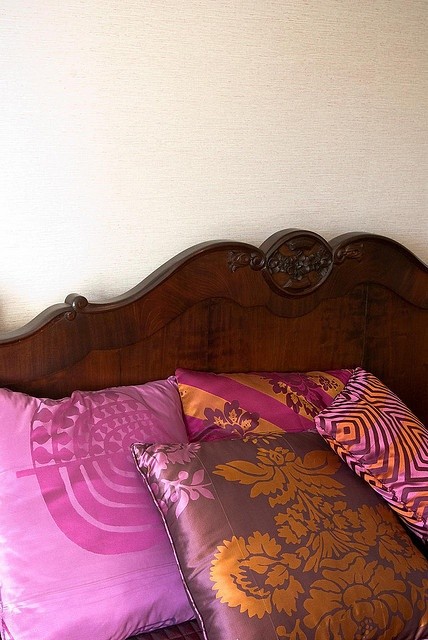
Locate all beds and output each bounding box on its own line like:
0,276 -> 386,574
0,228 -> 428,639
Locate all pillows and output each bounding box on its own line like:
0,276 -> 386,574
0,376 -> 195,640
313,366 -> 428,547
176,367 -> 353,443
130,429 -> 428,639
